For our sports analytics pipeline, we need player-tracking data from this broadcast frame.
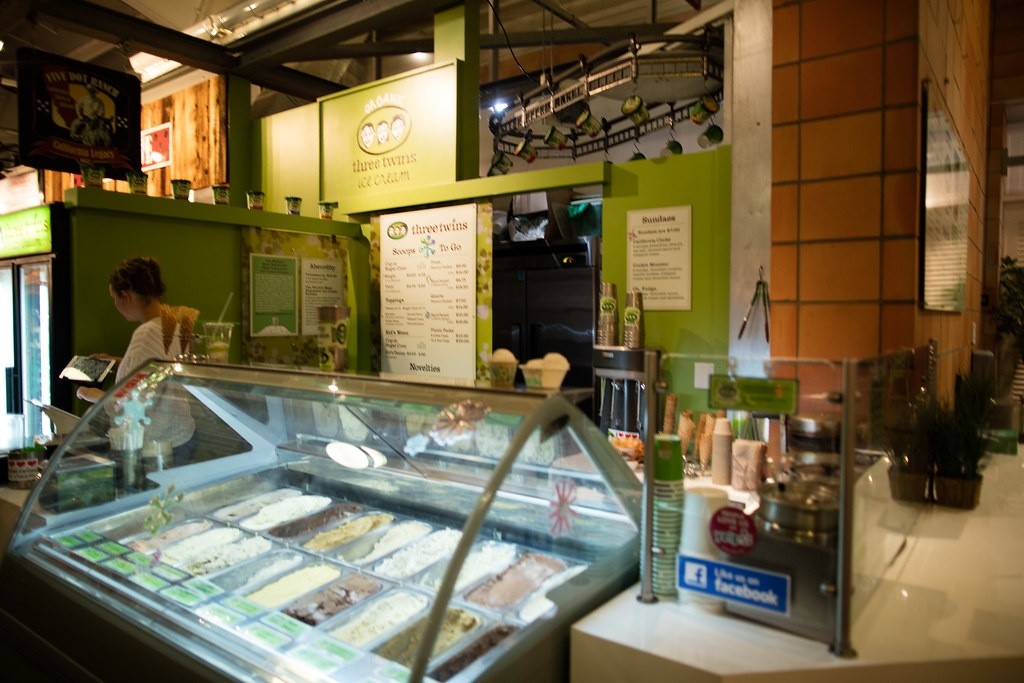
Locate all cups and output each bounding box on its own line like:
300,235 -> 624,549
622,290 -> 645,349
597,282 -> 620,346
648,433 -> 732,617
317,305 -> 351,372
202,321 -> 234,365
488,358 -> 519,388
107,447 -> 143,493
137,454 -> 173,492
732,440 -> 765,490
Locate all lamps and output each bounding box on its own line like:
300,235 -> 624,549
487,77 -> 723,178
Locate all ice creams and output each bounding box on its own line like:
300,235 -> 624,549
487,348 -> 518,388
519,352 -> 570,390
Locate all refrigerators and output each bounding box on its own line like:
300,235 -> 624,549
0,201 -> 69,455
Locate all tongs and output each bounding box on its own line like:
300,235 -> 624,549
738,280 -> 770,343
740,412 -> 761,440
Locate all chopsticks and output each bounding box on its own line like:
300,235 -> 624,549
105,418 -> 145,449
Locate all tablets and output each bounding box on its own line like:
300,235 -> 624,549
59,355 -> 116,382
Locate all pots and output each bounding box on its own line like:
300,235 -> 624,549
753,454 -> 848,536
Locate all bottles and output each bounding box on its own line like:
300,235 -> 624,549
712,418 -> 733,485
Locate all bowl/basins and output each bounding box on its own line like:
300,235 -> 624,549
520,365 -> 568,389
126,484 -> 591,674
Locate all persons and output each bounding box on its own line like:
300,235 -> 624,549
76,256 -> 198,466
